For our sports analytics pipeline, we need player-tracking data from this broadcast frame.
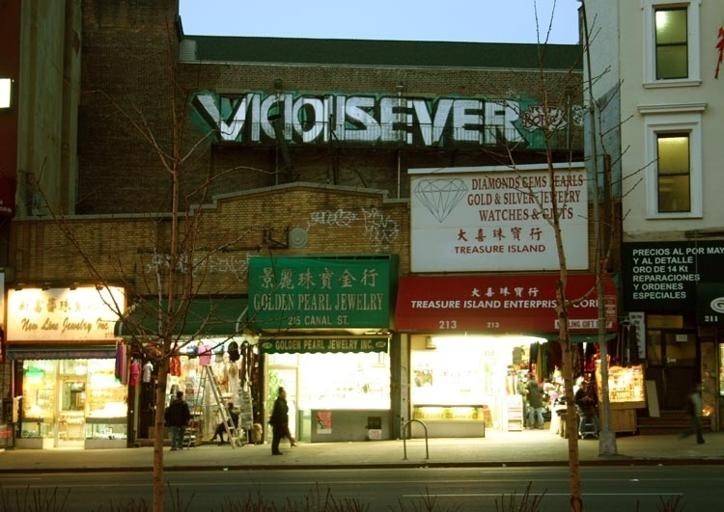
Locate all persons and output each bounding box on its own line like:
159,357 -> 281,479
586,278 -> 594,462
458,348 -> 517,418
170,391 -> 190,451
681,384 -> 705,444
209,403 -> 238,441
268,387 -> 297,455
575,382 -> 600,437
527,384 -> 550,430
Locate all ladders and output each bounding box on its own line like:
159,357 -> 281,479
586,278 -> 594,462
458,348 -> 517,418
187,366 -> 240,449
